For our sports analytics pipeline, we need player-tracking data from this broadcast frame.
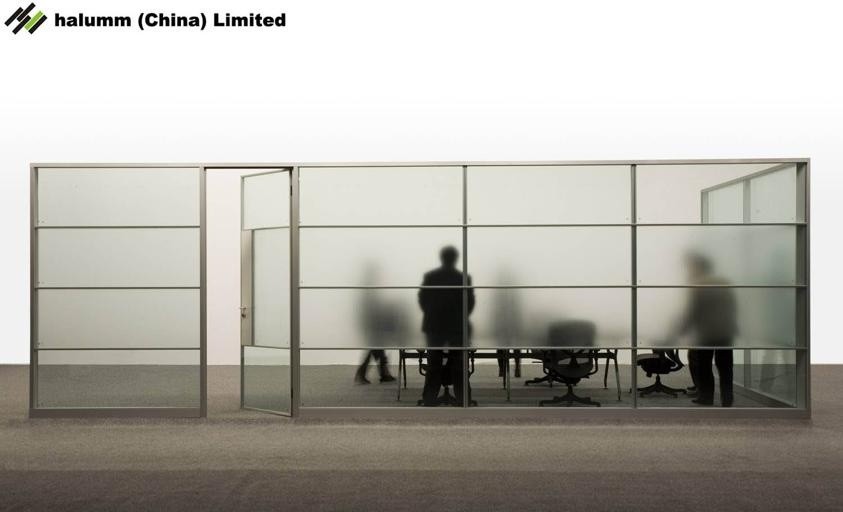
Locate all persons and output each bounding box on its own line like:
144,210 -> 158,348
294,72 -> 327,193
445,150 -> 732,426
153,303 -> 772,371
356,262 -> 395,384
417,246 -> 475,407
667,255 -> 738,407
686,350 -> 700,397
486,276 -> 521,378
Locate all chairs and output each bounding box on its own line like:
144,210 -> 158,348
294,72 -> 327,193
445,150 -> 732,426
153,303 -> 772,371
416,318 -> 687,407
